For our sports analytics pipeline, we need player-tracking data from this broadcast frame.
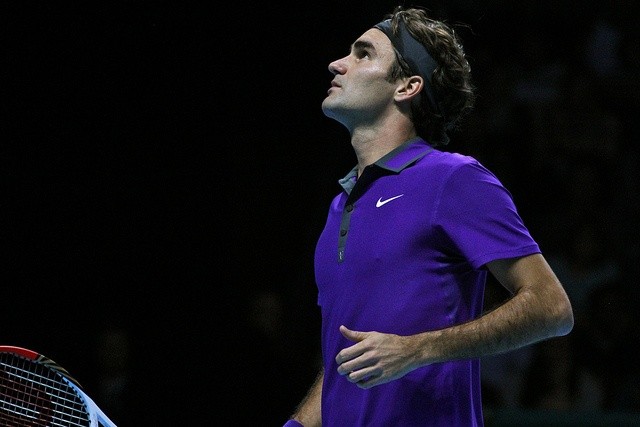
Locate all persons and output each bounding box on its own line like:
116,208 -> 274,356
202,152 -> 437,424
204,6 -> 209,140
284,7 -> 575,427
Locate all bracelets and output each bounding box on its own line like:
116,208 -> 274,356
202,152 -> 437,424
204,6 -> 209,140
282,418 -> 304,427
